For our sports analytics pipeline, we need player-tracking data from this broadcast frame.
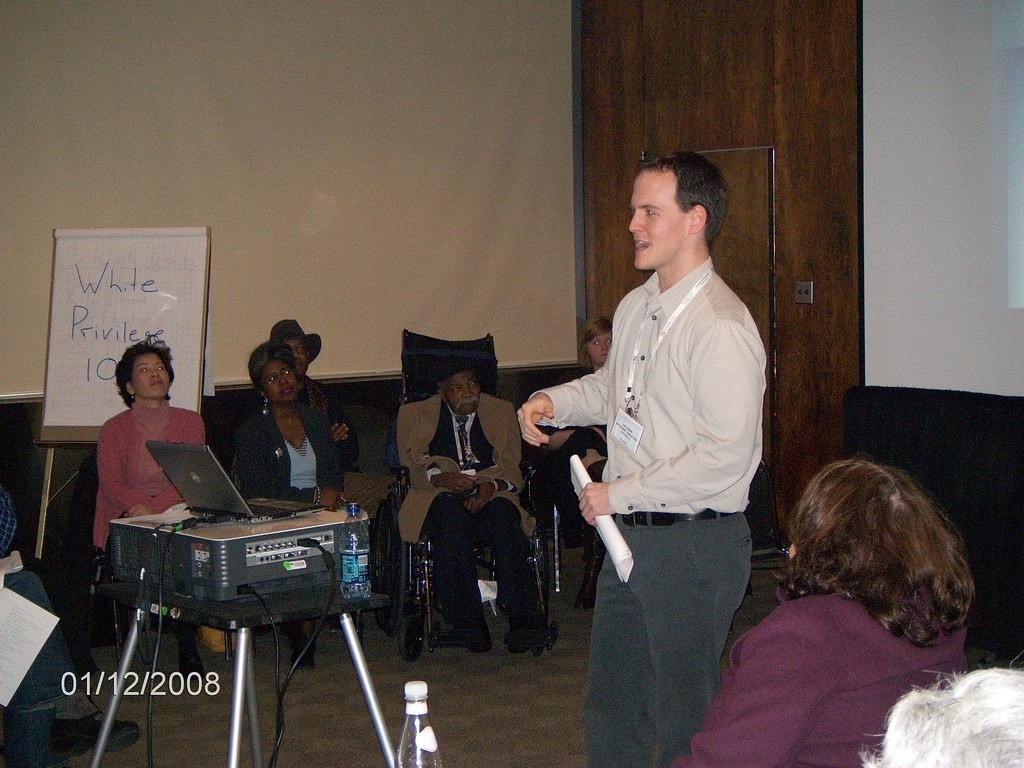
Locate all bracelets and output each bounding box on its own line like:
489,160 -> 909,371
315,485 -> 320,506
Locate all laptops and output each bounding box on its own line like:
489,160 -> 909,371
147,439 -> 326,524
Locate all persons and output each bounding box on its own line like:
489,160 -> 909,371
230,319 -> 360,669
668,457 -> 975,768
0,480 -> 141,768
865,669 -> 1024,768
518,150 -> 766,768
544,316 -> 613,608
396,355 -> 537,654
92,342 -> 206,681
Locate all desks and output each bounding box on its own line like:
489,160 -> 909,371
85,576 -> 401,768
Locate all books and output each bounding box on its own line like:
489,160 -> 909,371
0,551 -> 60,707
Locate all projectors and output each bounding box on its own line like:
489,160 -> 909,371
109,510 -> 371,603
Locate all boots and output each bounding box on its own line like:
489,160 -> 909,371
292,619 -> 316,669
575,531 -> 608,609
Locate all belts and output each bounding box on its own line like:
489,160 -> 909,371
622,508 -> 731,527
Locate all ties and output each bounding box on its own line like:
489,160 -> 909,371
456,416 -> 471,469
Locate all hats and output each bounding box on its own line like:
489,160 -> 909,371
270,320 -> 322,364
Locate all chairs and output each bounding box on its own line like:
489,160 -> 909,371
524,450 -> 581,594
65,451 -> 175,634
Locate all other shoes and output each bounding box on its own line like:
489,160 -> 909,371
47,719 -> 140,758
178,649 -> 204,681
450,609 -> 493,652
505,626 -> 542,652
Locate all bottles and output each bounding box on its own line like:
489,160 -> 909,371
339,503 -> 370,597
395,681 -> 442,768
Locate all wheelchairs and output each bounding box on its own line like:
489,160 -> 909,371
389,329 -> 555,662
